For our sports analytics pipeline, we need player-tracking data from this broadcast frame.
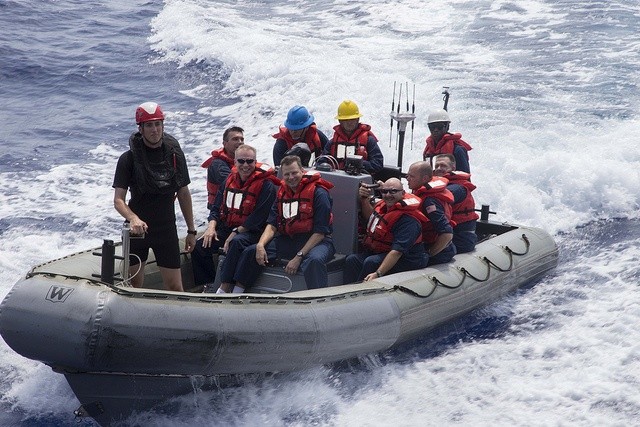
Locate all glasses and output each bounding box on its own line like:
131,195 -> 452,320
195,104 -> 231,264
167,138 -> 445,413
237,158 -> 254,164
382,189 -> 401,194
429,124 -> 445,130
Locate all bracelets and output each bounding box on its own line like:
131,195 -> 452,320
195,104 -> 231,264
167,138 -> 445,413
376,270 -> 383,278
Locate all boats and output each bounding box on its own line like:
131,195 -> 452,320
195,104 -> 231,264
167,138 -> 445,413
1,82 -> 560,426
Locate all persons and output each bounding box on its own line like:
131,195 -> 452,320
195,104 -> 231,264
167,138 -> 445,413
232,155 -> 335,295
195,144 -> 275,296
423,108 -> 471,188
343,177 -> 428,283
272,107 -> 331,169
434,154 -> 479,254
113,101 -> 197,292
406,161 -> 456,263
201,126 -> 244,223
316,101 -> 384,176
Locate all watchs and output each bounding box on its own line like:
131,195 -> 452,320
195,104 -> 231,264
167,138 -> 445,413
425,248 -> 432,256
232,228 -> 239,234
187,231 -> 197,235
296,251 -> 304,258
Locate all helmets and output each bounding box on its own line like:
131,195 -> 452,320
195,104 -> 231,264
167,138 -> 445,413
285,105 -> 314,129
426,109 -> 451,124
337,100 -> 360,119
136,102 -> 165,123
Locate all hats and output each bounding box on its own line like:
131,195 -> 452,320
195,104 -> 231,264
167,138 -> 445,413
285,142 -> 311,163
